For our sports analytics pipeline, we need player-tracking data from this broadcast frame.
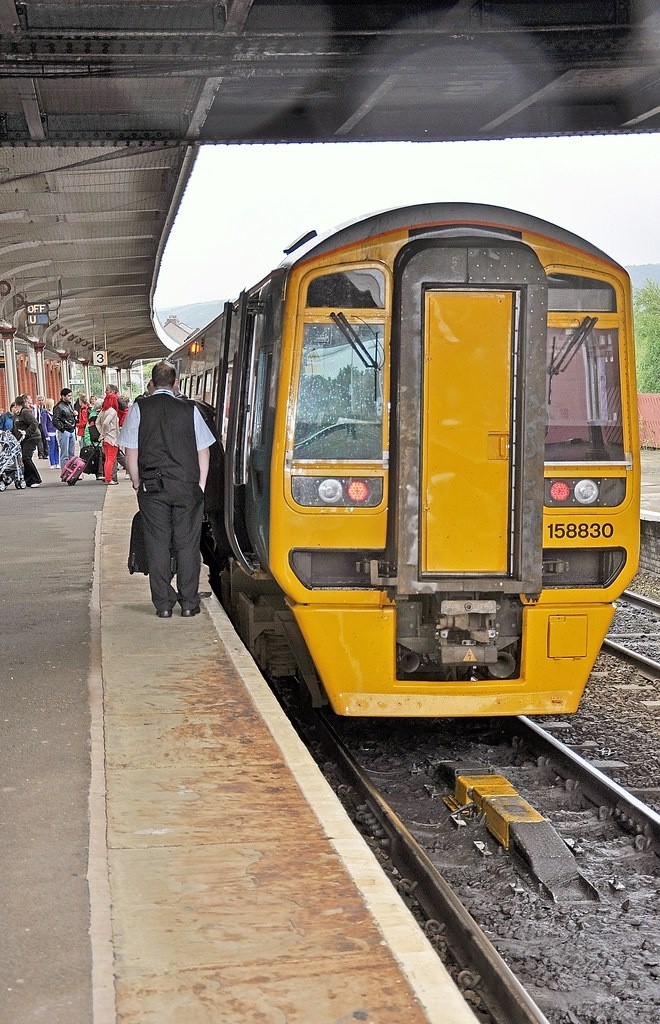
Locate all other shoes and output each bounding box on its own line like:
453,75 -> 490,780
157,604 -> 172,618
105,479 -> 117,485
50,465 -> 61,469
179,600 -> 201,618
31,482 -> 42,487
124,473 -> 131,479
38,453 -> 48,459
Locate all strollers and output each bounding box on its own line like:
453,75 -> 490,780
0,428 -> 27,489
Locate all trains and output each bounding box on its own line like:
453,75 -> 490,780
166,201 -> 642,723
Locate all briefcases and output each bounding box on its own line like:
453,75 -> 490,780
128,508 -> 178,578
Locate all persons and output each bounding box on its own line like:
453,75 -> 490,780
20,393 -> 49,459
143,379 -> 225,531
74,396 -> 90,449
51,387 -> 83,481
0,402 -> 17,431
88,395 -> 98,410
72,391 -> 85,424
116,359 -> 217,618
12,396 -> 43,488
40,398 -> 60,469
96,394 -> 120,485
105,384 -> 133,427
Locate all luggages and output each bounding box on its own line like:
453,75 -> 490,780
79,440 -> 102,475
60,438 -> 104,485
